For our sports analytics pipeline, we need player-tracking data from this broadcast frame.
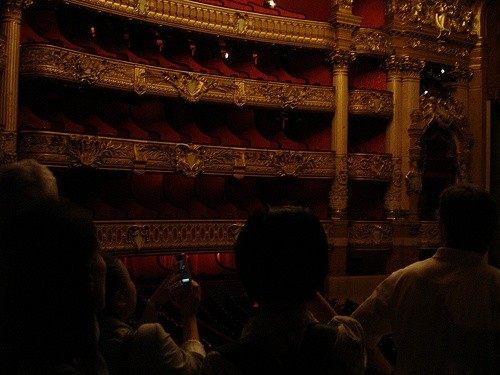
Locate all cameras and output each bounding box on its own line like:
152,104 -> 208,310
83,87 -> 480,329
181,277 -> 190,283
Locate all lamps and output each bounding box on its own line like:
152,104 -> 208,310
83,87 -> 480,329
90,10 -> 260,65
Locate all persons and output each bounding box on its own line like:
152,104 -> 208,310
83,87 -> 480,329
201,205 -> 367,375
351,185 -> 500,375
102,252 -> 207,375
0,159 -> 107,375
